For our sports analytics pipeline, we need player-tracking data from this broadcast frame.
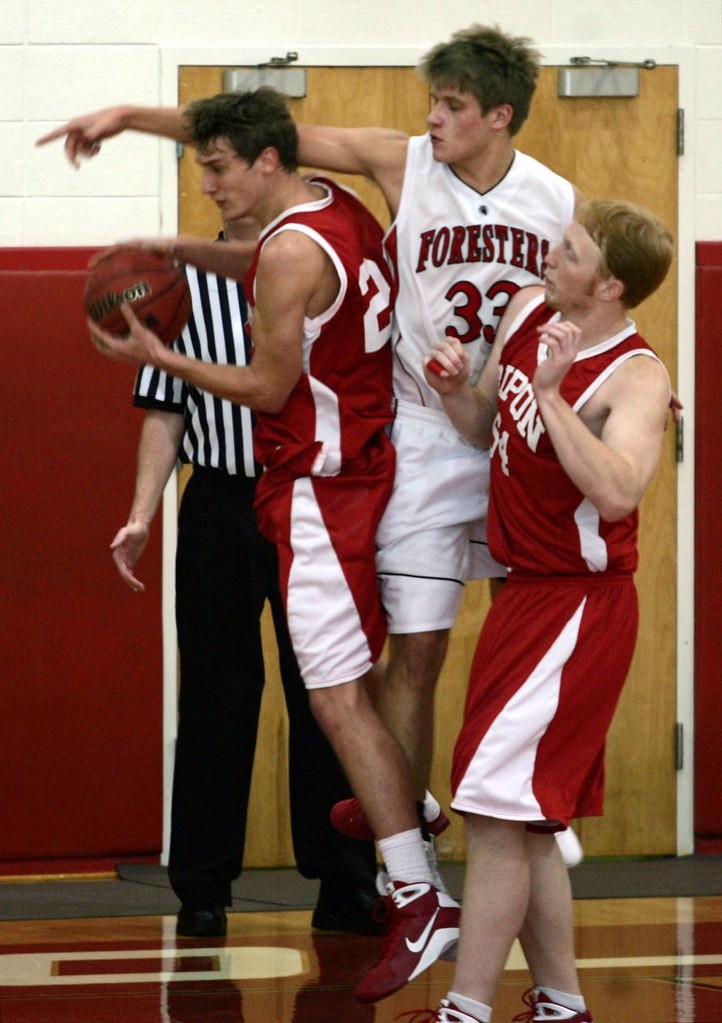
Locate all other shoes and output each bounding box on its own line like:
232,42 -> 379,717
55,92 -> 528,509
423,843 -> 446,891
176,903 -> 229,938
308,887 -> 386,938
554,826 -> 583,868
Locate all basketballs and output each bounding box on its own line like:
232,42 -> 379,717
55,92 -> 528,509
86,246 -> 190,346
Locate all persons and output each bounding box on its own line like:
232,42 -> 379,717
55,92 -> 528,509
422,196 -> 672,1022
84,87 -> 461,999
34,25 -> 685,872
106,210 -> 391,936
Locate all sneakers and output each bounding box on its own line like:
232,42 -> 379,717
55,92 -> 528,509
392,999 -> 486,1023
512,985 -> 593,1023
330,785 -> 451,838
353,880 -> 460,1003
